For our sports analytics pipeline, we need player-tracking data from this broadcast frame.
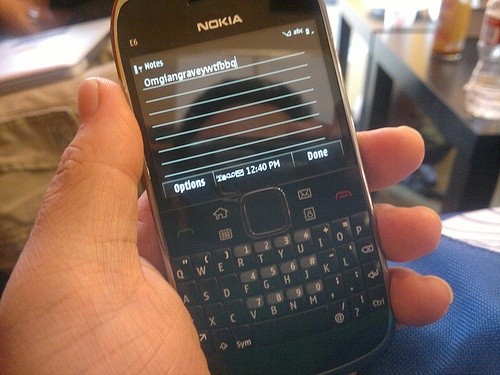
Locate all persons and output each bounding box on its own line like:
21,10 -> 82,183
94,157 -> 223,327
0,78 -> 455,375
177,77 -> 331,147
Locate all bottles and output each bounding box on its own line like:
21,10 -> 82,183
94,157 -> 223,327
461,0 -> 500,120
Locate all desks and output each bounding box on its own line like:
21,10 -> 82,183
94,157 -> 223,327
339,10 -> 499,213
1,14 -> 110,92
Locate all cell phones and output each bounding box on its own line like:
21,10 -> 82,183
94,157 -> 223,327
109,0 -> 397,375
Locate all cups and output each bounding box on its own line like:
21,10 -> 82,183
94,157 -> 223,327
425,0 -> 471,62
